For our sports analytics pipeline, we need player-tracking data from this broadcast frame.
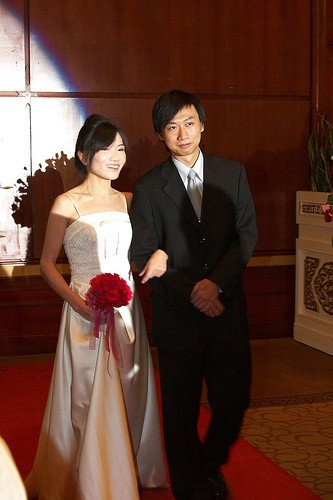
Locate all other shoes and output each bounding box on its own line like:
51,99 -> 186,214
170,467 -> 229,500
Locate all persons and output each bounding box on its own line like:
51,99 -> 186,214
127,89 -> 258,500
39,113 -> 168,500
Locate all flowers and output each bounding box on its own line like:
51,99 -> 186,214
84,273 -> 133,379
319,195 -> 333,223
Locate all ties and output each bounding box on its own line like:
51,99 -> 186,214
186,169 -> 203,222
